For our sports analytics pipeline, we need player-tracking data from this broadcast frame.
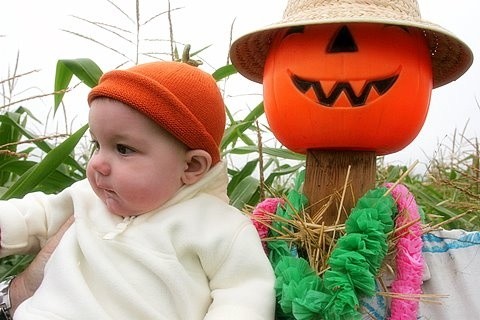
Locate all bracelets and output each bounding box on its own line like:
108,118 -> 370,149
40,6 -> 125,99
0,274 -> 16,320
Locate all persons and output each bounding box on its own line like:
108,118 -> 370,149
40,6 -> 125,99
230,1 -> 480,320
0,61 -> 277,320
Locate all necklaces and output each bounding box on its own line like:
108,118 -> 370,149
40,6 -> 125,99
253,182 -> 424,320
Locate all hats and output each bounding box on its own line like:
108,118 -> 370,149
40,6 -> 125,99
229,0 -> 474,89
88,61 -> 226,168
